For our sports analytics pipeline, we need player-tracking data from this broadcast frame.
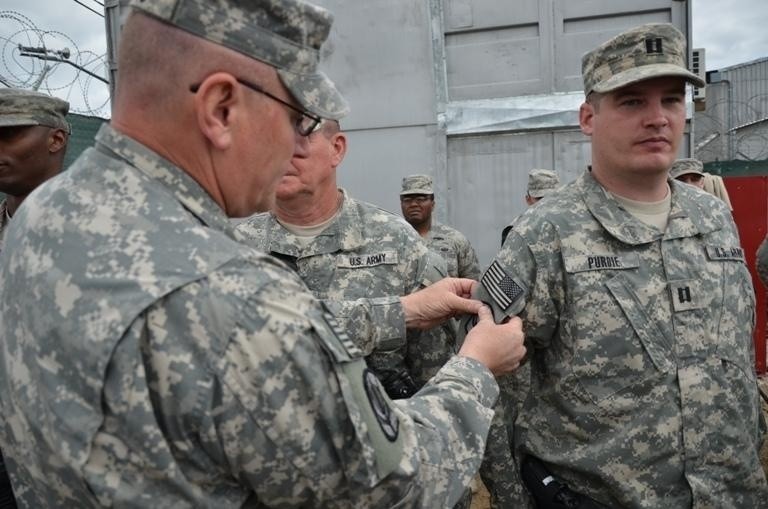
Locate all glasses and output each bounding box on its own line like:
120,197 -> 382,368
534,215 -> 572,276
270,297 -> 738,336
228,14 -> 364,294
189,73 -> 322,136
399,195 -> 432,202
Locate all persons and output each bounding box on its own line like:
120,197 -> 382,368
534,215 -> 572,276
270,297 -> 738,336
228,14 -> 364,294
399,173 -> 483,349
0,0 -> 532,508
463,19 -> 767,509
225,114 -> 450,403
1,87 -> 71,234
498,158 -> 557,238
667,159 -> 708,191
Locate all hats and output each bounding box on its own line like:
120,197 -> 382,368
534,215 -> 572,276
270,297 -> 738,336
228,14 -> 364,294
579,23 -> 708,103
669,157 -> 706,181
128,0 -> 350,122
526,168 -> 560,200
398,174 -> 435,197
1,87 -> 72,137
